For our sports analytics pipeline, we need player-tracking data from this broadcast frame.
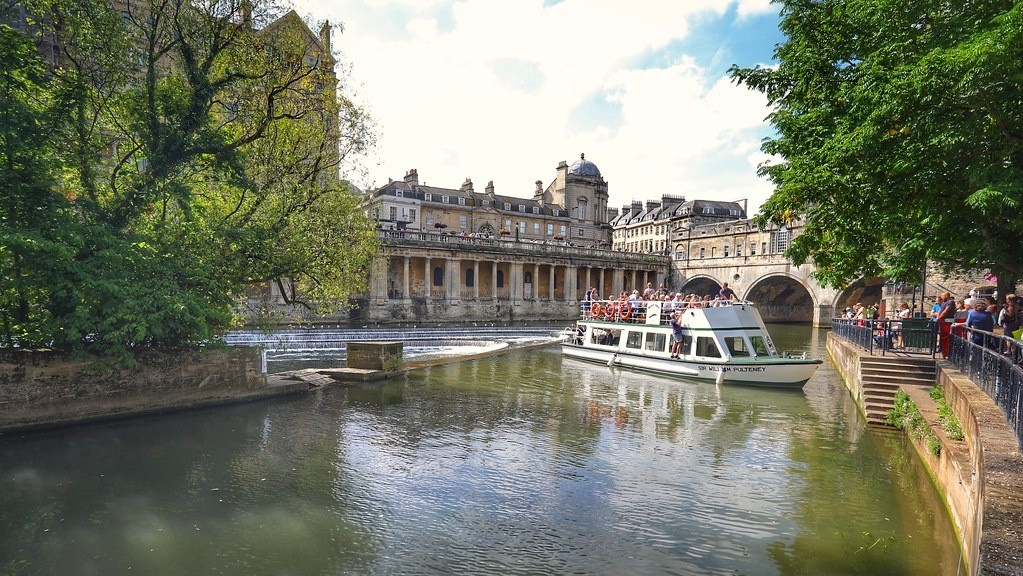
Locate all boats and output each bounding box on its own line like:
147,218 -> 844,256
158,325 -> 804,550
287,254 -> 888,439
560,299 -> 823,388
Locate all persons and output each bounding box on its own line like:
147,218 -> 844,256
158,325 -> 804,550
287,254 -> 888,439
422,227 -> 427,240
873,303 -> 910,350
591,333 -> 594,343
928,287 -> 997,378
401,227 -> 412,238
832,302 -> 879,340
583,287 -> 602,321
670,312 -> 683,360
998,295 -> 1023,407
461,231 -> 489,244
542,240 -> 554,245
604,282 -> 740,324
566,242 -> 571,247
529,238 -> 537,243
600,330 -> 613,346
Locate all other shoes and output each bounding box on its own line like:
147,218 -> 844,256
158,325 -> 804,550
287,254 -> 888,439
671,355 -> 681,359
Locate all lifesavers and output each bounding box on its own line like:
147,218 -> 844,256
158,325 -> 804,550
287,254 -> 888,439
604,303 -> 616,317
591,303 -> 601,317
620,303 -> 630,318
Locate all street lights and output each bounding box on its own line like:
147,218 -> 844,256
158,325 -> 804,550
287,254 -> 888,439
515,222 -> 519,240
434,222 -> 447,241
